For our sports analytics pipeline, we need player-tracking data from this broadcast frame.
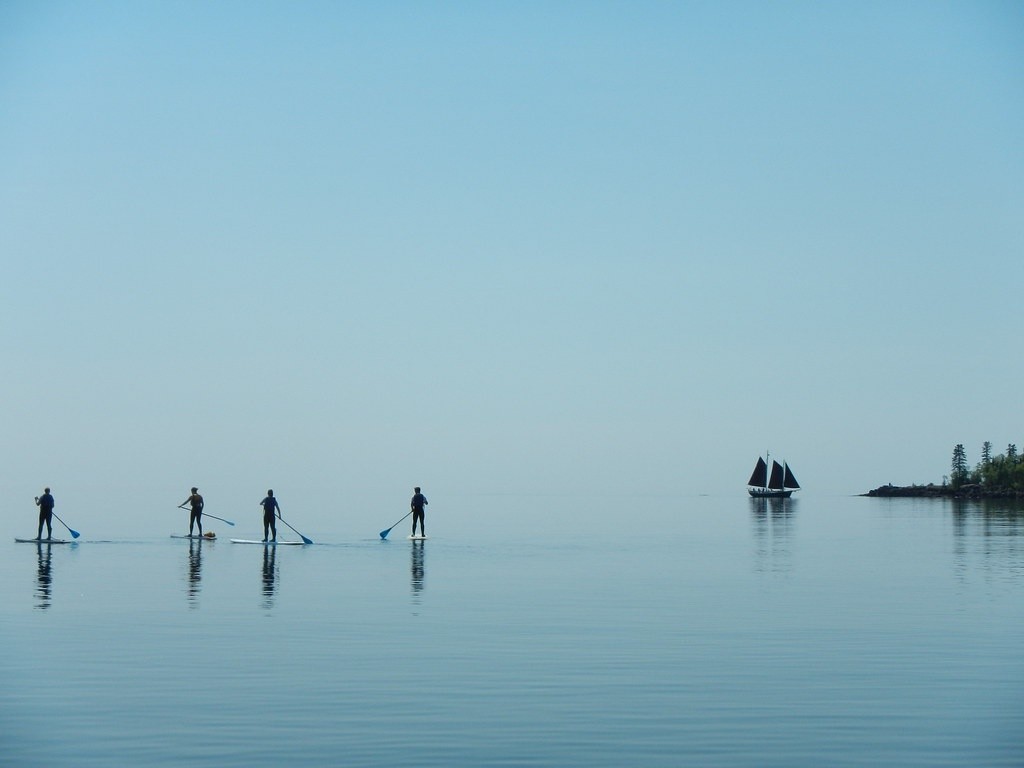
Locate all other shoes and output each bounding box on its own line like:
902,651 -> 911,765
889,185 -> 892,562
411,534 -> 416,538
421,534 -> 426,537
261,539 -> 268,542
184,534 -> 192,537
198,534 -> 202,537
269,538 -> 276,542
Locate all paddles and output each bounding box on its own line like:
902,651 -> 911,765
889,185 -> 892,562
50,510 -> 80,540
179,505 -> 236,527
379,509 -> 418,539
264,507 -> 315,543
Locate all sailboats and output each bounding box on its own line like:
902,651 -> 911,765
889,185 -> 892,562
745,449 -> 801,499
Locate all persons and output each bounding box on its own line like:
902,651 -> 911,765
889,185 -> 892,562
34,488 -> 54,541
260,489 -> 281,542
411,487 -> 428,537
178,487 -> 204,537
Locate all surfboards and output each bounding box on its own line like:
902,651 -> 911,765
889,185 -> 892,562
14,535 -> 72,544
230,538 -> 315,545
170,534 -> 216,540
407,533 -> 427,540
